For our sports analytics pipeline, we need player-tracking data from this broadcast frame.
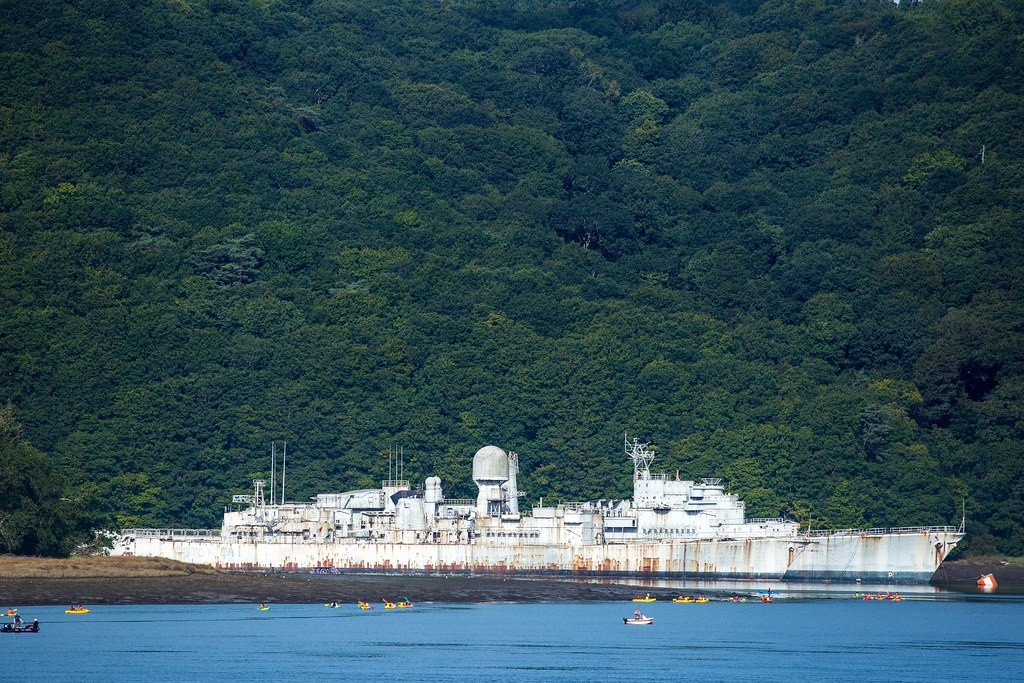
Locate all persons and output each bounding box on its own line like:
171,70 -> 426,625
70,605 -> 82,612
358,598 -> 369,607
634,608 -> 641,620
260,602 -> 264,608
678,594 -> 684,599
7,607 -> 18,614
14,614 -> 25,627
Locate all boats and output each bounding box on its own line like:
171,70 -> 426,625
384,603 -> 397,609
0,619 -> 41,632
329,604 -> 341,609
623,616 -> 655,625
760,597 -> 774,603
362,606 -> 372,610
0,612 -> 20,617
695,598 -> 710,604
632,598 -> 657,604
258,605 -> 271,611
673,598 -> 696,603
399,603 -> 414,608
852,592 -> 904,602
66,608 -> 91,614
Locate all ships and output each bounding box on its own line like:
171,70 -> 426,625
76,426 -> 970,599
